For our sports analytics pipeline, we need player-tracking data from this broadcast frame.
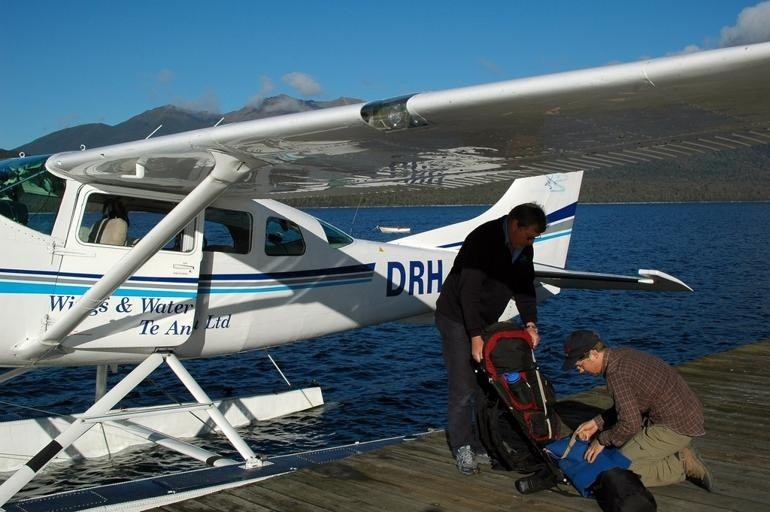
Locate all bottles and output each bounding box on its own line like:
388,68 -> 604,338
461,445 -> 474,476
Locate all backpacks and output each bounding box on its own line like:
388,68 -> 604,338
473,321 -> 567,493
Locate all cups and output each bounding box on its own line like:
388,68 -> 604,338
504,371 -> 533,406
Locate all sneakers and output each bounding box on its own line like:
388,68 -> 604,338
677,446 -> 715,493
455,443 -> 480,475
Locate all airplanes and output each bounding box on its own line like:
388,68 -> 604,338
0,41 -> 770,512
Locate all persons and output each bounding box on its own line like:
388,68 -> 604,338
433,202 -> 547,478
562,328 -> 715,495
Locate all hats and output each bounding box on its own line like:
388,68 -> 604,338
558,328 -> 600,373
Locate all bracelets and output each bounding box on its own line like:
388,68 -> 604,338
527,325 -> 537,330
